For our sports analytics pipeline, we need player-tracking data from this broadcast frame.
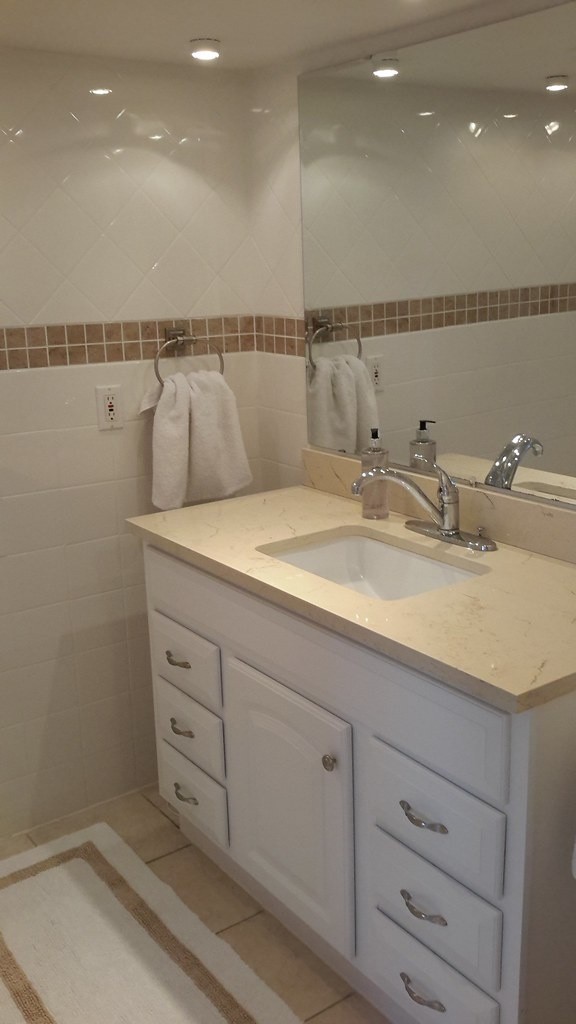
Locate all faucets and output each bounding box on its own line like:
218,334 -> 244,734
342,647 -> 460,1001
351,468 -> 461,533
483,434 -> 545,488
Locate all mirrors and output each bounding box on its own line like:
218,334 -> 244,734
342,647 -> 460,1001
298,0 -> 576,506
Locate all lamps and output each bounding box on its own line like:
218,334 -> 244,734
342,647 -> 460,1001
190,40 -> 221,61
370,55 -> 401,79
545,75 -> 571,92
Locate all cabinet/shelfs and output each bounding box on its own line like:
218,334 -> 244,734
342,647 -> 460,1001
124,543 -> 576,1023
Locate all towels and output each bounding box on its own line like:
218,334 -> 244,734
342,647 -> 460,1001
305,355 -> 381,454
138,370 -> 253,510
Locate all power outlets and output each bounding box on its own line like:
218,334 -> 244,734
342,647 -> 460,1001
366,354 -> 385,393
95,385 -> 124,431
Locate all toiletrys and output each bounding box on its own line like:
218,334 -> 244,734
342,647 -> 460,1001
410,419 -> 437,470
360,429 -> 390,519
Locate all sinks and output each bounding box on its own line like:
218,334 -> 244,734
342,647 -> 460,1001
484,433 -> 576,502
253,523 -> 496,612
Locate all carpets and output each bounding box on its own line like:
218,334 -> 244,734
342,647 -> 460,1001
0,821 -> 303,1024
